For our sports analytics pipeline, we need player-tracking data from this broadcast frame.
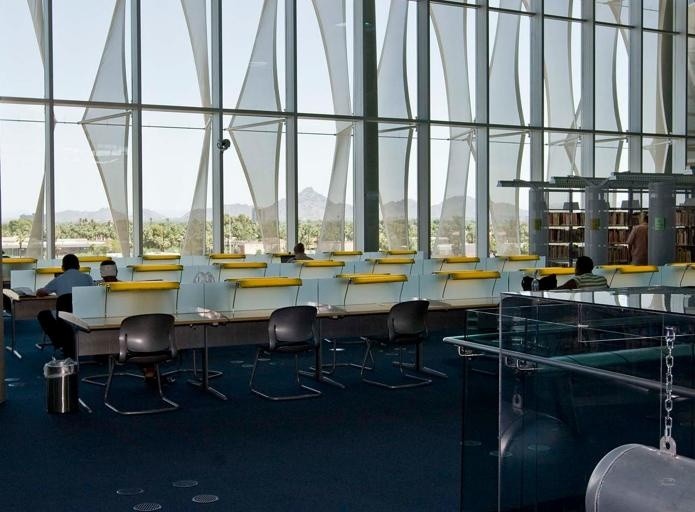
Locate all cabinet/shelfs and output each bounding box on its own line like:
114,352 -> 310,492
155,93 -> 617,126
545,206 -> 695,264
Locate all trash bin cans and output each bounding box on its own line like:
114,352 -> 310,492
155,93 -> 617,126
43,356 -> 77,414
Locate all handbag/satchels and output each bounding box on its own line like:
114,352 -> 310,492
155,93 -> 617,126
521,274 -> 557,291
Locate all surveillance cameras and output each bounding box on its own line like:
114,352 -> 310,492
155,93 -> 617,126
216,139 -> 230,151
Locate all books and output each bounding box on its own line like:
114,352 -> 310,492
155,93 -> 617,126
548,228 -> 584,242
609,229 -> 631,242
609,246 -> 635,264
677,248 -> 695,264
675,229 -> 695,245
547,213 -> 585,225
608,212 -> 648,225
548,262 -> 569,267
676,209 -> 695,226
548,245 -> 584,259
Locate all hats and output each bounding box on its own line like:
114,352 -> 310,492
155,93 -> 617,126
100,264 -> 117,277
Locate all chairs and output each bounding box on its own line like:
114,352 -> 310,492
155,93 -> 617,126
473,297 -> 534,379
249,305 -> 322,400
52,293 -> 107,368
360,300 -> 434,390
103,314 -> 180,415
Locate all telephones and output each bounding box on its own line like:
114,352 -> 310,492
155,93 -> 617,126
12,286 -> 36,296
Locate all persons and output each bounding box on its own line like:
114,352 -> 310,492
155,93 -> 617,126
94,260 -> 123,285
626,213 -> 648,266
36,254 -> 93,356
286,243 -> 316,263
557,256 -> 609,289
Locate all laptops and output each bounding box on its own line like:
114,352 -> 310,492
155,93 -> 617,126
280,255 -> 295,263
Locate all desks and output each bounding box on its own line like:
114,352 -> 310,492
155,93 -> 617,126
57,310 -> 228,415
334,298 -> 452,390
217,304 -> 345,401
1,288 -> 58,358
439,293 -> 500,378
